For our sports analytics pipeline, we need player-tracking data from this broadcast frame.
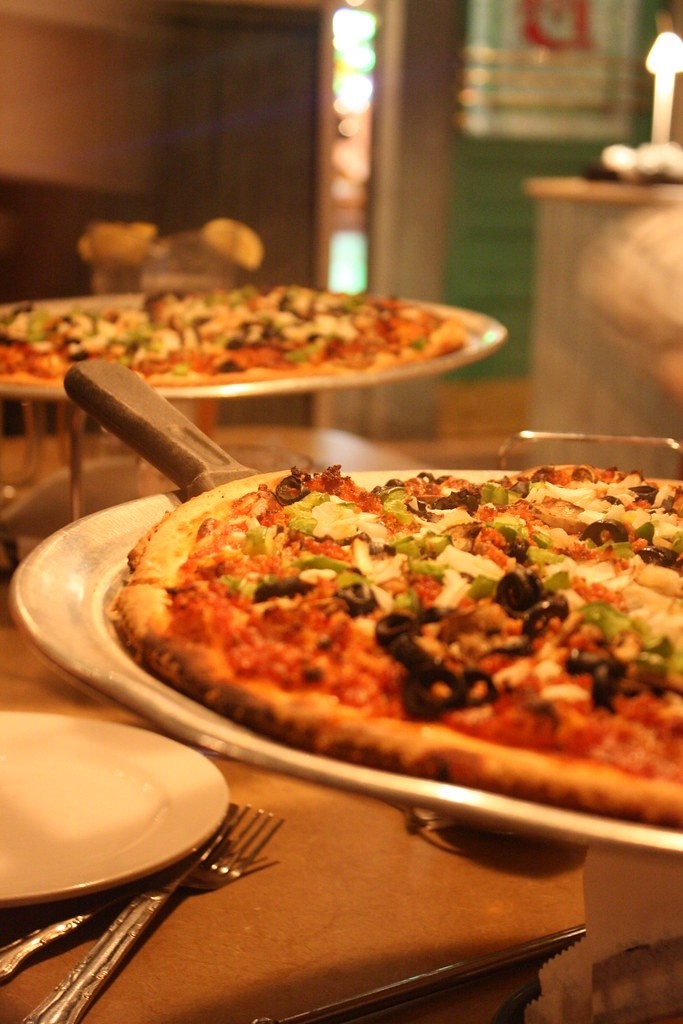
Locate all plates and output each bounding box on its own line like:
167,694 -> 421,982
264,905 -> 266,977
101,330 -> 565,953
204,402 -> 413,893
2,295 -> 507,398
0,710 -> 230,908
11,468 -> 682,853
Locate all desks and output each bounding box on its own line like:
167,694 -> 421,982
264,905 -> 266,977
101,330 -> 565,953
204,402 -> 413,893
1,427 -> 591,1024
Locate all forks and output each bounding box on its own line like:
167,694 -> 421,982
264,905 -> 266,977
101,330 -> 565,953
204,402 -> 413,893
0,803 -> 285,1024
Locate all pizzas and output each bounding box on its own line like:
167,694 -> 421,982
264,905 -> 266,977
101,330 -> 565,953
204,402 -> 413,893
0,283 -> 474,389
112,466 -> 683,830
